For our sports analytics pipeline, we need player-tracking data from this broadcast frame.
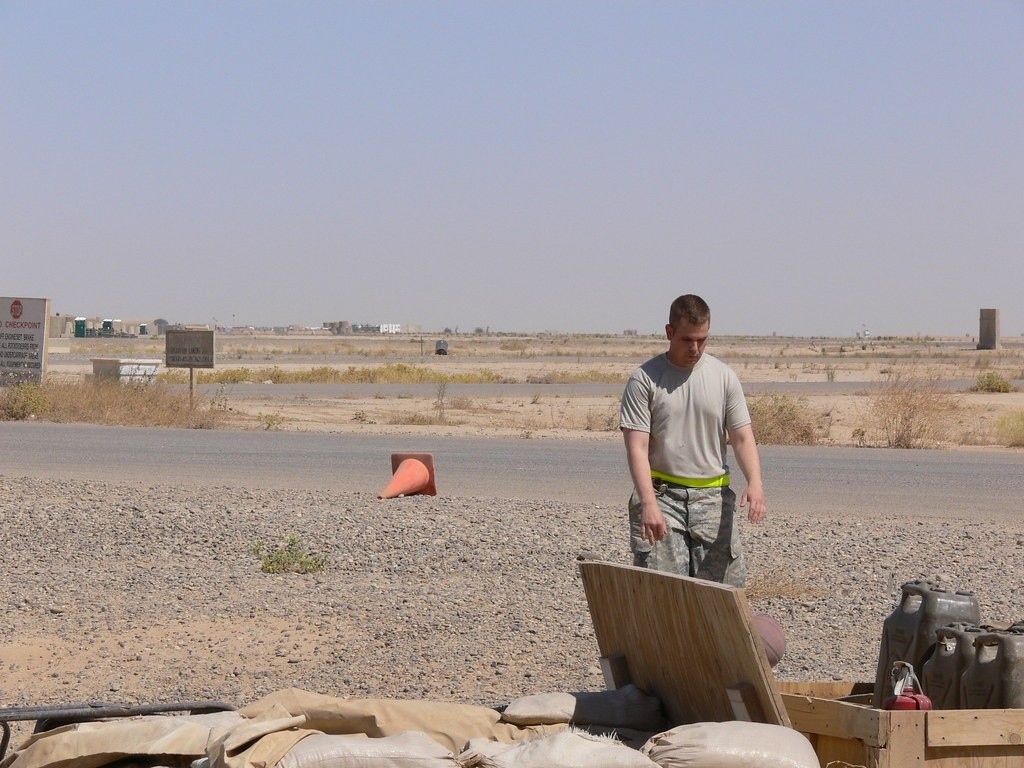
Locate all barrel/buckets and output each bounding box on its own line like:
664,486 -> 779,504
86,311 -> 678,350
871,579 -> 981,706
435,340 -> 448,355
919,622 -> 994,712
958,626 -> 1024,710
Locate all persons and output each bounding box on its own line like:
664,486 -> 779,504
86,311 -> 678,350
620,294 -> 766,588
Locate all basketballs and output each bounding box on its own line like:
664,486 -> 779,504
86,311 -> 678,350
751,613 -> 788,667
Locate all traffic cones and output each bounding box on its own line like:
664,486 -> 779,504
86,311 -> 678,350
377,452 -> 435,498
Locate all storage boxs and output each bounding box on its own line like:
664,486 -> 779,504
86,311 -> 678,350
780,681 -> 1024,768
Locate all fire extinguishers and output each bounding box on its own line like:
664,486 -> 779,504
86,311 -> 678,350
882,660 -> 933,710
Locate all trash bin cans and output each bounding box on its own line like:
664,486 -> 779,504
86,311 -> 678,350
435,340 -> 448,355
139,323 -> 147,334
102,318 -> 113,337
75,317 -> 87,337
